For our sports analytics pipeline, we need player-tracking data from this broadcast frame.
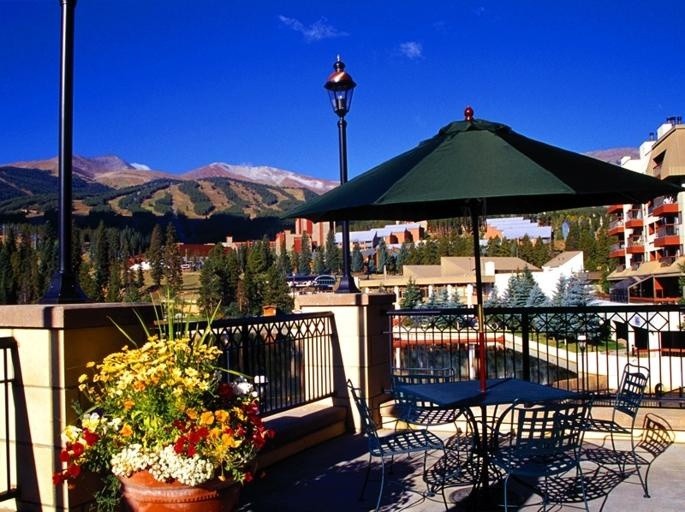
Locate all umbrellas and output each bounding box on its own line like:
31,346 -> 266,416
281,119 -> 685,489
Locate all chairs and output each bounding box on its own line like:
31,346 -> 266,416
486,389 -> 597,512
389,365 -> 471,495
554,362 -> 652,497
342,378 -> 451,512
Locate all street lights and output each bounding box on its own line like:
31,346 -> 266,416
323,49 -> 360,294
578,336 -> 589,392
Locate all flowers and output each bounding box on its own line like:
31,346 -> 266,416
52,282 -> 278,492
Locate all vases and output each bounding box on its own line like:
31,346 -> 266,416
113,471 -> 244,512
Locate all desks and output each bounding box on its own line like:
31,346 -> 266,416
390,376 -> 595,512
